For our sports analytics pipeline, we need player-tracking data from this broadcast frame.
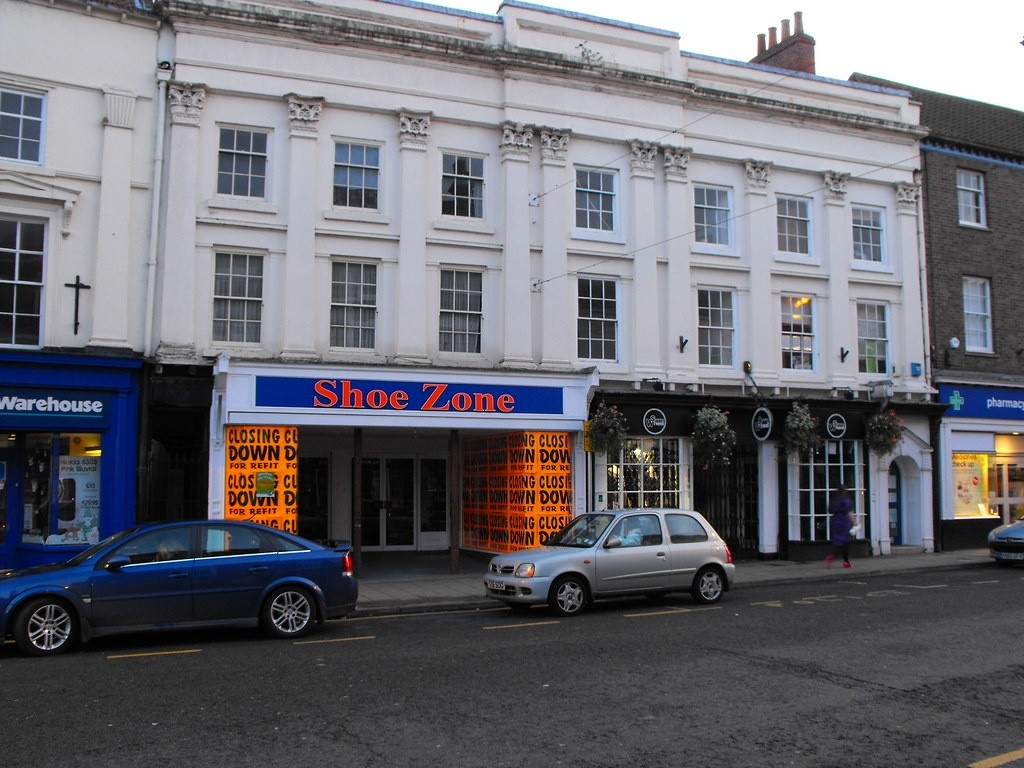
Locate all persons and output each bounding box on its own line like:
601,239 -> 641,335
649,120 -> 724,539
606,516 -> 644,547
827,484 -> 861,568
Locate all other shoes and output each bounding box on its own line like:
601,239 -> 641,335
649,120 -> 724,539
843,561 -> 852,568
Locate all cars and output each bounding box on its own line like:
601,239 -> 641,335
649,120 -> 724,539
988,514 -> 1024,568
483,505 -> 737,617
0,517 -> 358,656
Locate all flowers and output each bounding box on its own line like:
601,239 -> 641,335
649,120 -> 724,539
588,403 -> 630,458
684,404 -> 738,473
774,401 -> 827,466
863,409 -> 908,459
